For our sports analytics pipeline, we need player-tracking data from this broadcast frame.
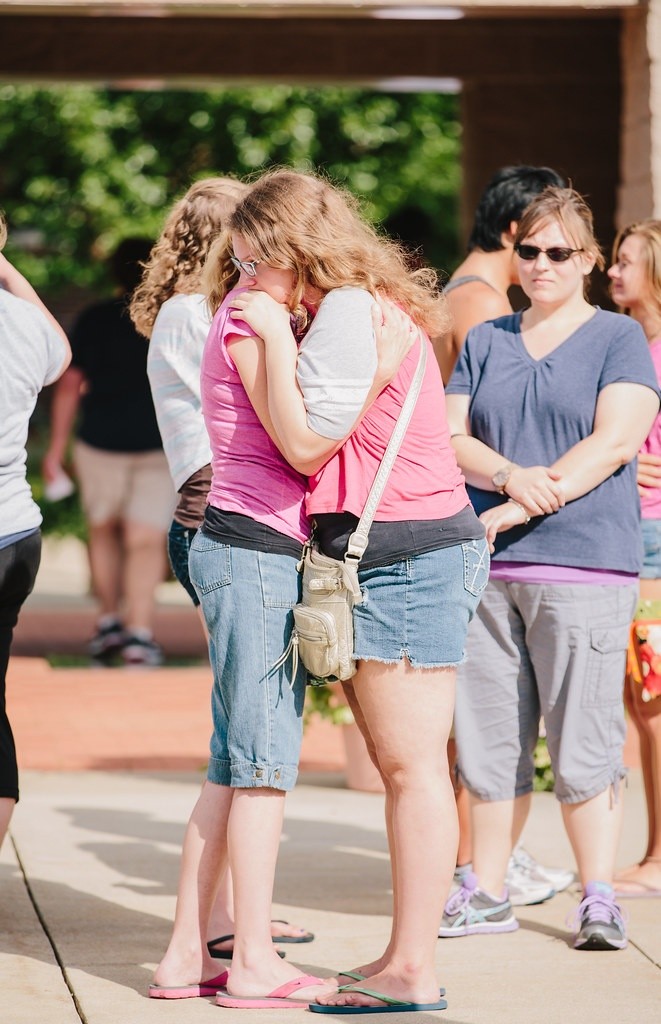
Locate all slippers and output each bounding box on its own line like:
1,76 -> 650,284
149,962 -> 232,1000
612,876 -> 661,896
269,917 -> 314,943
217,969 -> 338,1009
208,932 -> 285,960
308,988 -> 448,1015
335,965 -> 446,996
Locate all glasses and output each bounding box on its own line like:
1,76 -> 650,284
231,253 -> 263,277
513,241 -> 584,262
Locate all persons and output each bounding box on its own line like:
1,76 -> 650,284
227,164 -> 491,1014
429,163 -> 660,907
438,179 -> 661,952
43,235 -> 182,670
0,208 -> 71,852
129,176 -> 421,1010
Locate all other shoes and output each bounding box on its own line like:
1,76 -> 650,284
88,623 -> 167,666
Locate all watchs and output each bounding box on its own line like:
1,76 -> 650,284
492,465 -> 523,495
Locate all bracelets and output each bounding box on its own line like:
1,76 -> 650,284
508,498 -> 531,525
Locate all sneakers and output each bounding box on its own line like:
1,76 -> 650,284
434,873 -> 519,938
513,843 -> 575,891
564,879 -> 627,949
504,856 -> 553,906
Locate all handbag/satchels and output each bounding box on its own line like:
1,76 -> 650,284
269,532 -> 362,690
